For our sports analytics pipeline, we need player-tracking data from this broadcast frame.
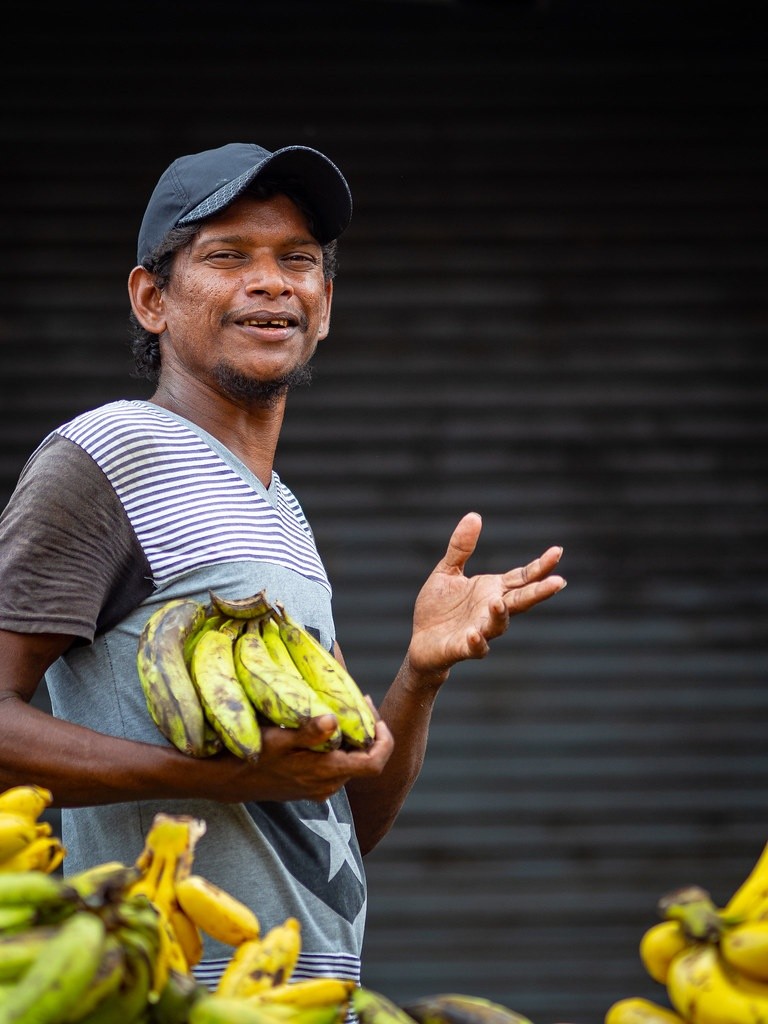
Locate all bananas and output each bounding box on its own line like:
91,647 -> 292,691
136,588 -> 376,767
0,785 -> 537,1024
605,838 -> 768,1024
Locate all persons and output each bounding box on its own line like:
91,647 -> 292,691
0,144 -> 568,1024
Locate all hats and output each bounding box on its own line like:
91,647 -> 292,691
137,143 -> 353,267
